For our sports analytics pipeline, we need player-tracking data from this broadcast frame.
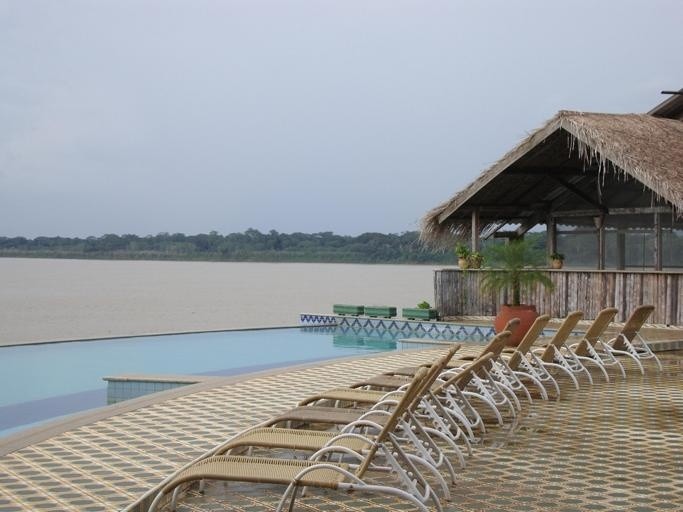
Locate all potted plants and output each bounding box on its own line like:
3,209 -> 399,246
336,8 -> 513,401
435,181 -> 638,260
550,251 -> 565,269
467,249 -> 484,269
480,232 -> 554,347
455,242 -> 470,269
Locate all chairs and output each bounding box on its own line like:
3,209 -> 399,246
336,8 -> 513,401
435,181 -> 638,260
334,305 -> 660,426
148,344 -> 503,512
